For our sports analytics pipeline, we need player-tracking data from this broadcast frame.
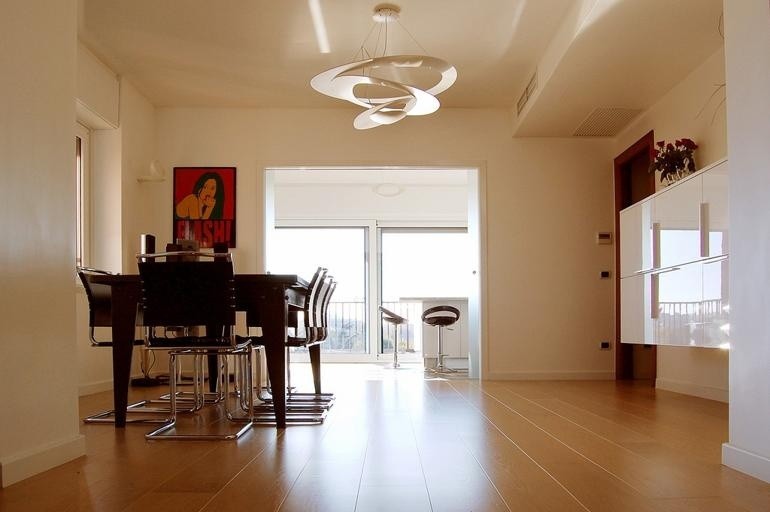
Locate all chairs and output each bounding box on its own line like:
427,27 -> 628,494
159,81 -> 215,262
77,267 -> 226,424
132,250 -> 250,442
235,311 -> 299,395
251,267 -> 335,426
378,306 -> 460,374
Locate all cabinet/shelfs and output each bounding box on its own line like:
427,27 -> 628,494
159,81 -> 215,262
619,158 -> 729,350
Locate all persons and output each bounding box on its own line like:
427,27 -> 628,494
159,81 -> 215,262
175,171 -> 224,222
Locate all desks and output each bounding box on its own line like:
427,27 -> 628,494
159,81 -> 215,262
90,273 -> 322,431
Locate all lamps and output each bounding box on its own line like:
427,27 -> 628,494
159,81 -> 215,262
308,8 -> 458,131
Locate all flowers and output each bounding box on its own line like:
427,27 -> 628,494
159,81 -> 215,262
648,136 -> 697,185
